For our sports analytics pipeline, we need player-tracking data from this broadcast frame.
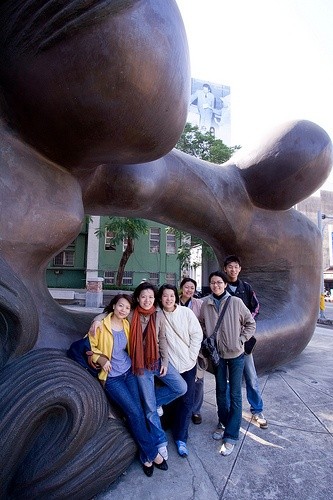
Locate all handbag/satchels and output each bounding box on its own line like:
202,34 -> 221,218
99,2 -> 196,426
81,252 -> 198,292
201,334 -> 220,368
68,336 -> 101,378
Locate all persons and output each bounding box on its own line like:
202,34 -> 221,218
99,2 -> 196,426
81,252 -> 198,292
178,278 -> 204,424
82,294 -> 168,478
157,283 -> 203,456
223,256 -> 268,428
91,281 -> 187,461
200,271 -> 256,456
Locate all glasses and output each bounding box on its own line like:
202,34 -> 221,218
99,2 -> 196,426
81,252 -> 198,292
210,281 -> 223,285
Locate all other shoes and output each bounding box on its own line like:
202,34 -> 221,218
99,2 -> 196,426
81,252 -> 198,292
212,422 -> 226,440
253,412 -> 268,429
143,463 -> 154,477
219,441 -> 235,457
152,452 -> 168,471
158,446 -> 168,460
191,412 -> 203,424
175,439 -> 188,457
